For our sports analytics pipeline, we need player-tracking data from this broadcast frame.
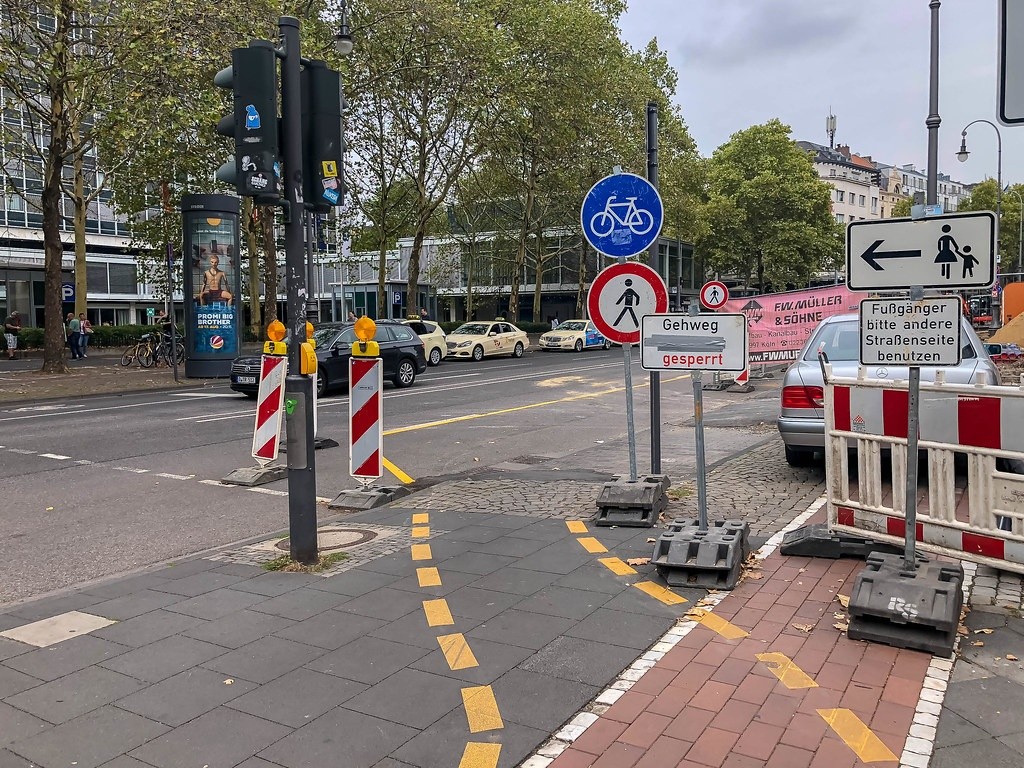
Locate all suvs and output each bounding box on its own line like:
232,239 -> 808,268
229,318 -> 429,398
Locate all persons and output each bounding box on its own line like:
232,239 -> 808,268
67,312 -> 84,360
551,316 -> 558,330
348,312 -> 358,322
76,313 -> 91,358
195,255 -> 232,307
157,310 -> 172,342
4,311 -> 23,360
421,307 -> 430,320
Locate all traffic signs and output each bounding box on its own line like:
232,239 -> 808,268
842,209 -> 996,291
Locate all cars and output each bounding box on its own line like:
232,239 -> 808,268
776,307 -> 1003,466
444,317 -> 530,362
398,314 -> 448,366
539,319 -> 612,352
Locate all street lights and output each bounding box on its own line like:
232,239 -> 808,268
956,120 -> 1002,339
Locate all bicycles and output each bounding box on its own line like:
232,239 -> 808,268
121,331 -> 184,368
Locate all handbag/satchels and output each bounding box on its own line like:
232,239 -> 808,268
84,320 -> 94,335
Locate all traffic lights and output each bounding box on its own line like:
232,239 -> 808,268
211,39 -> 276,200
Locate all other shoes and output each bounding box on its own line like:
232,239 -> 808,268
76,354 -> 79,357
79,357 -> 83,360
84,354 -> 87,357
69,358 -> 77,361
9,355 -> 19,360
